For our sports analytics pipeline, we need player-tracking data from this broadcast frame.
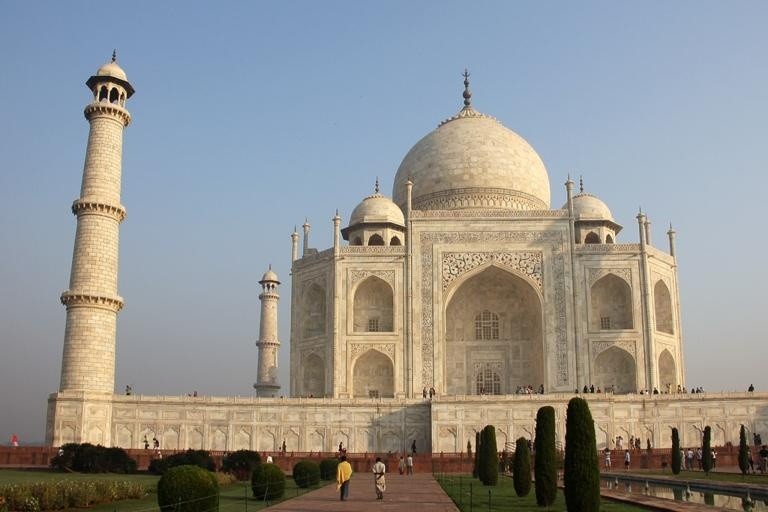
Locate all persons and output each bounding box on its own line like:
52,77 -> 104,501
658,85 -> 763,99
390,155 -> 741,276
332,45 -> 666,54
748,384 -> 754,392
602,433 -> 768,475
125,385 -> 132,395
267,441 -> 287,465
144,435 -> 163,459
422,387 -> 436,398
335,439 -> 417,476
372,457 -> 386,499
515,384 -> 704,394
528,440 -> 531,451
335,456 -> 353,501
